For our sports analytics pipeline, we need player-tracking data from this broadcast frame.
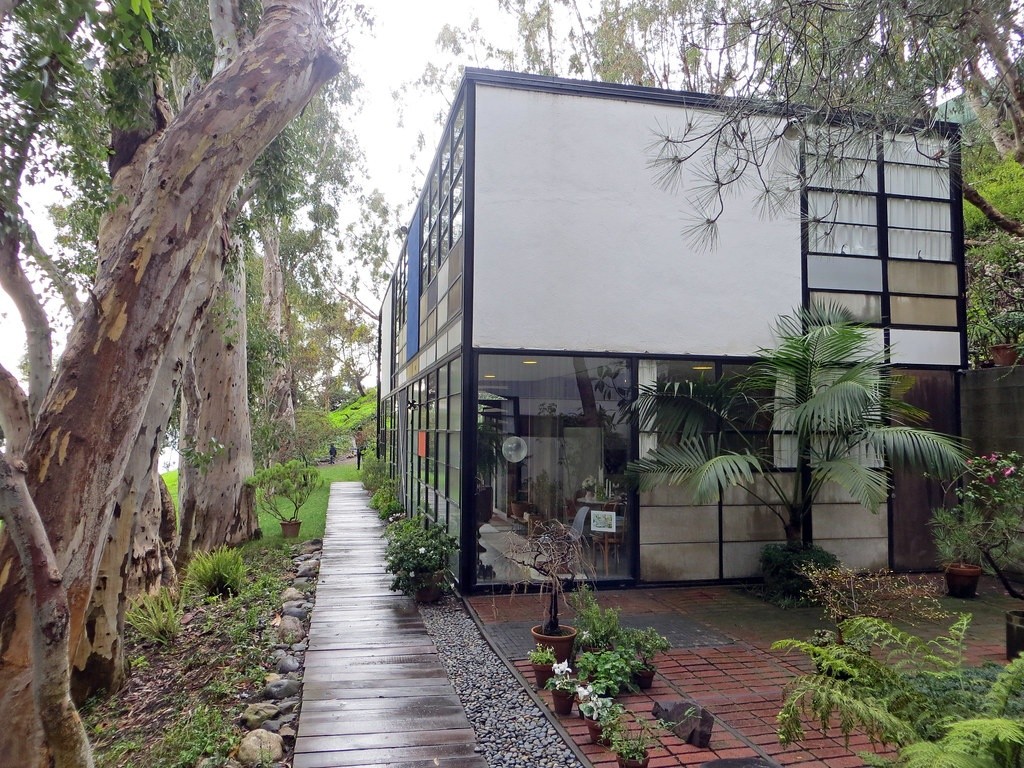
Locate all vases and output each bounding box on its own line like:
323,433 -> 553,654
585,491 -> 593,501
492,518 -> 598,663
551,688 -> 578,714
414,568 -> 445,602
943,565 -> 981,598
584,715 -> 620,745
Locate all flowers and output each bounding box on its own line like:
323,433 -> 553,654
576,685 -> 624,720
385,522 -> 458,601
543,659 -> 581,694
581,475 -> 597,492
941,451 -> 1021,568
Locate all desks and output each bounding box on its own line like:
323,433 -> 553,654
576,496 -> 627,578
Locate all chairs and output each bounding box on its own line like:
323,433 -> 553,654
539,506 -> 590,574
590,500 -> 627,577
511,504 -> 537,536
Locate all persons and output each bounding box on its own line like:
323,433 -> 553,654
328,444 -> 336,464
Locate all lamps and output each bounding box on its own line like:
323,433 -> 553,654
783,116 -> 805,141
501,436 -> 528,463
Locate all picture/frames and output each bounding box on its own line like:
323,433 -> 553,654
590,510 -> 616,532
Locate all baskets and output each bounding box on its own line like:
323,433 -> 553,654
511,500 -> 528,517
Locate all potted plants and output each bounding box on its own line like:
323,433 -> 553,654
571,584 -> 622,652
243,460 -> 325,538
576,647 -> 640,698
378,500 -> 404,523
966,265 -> 1024,367
596,703 -> 703,768
526,643 -> 557,688
928,504 -> 1024,662
792,558 -> 957,680
182,542 -> 245,598
615,626 -> 673,688
124,587 -> 185,645
625,296 -> 983,605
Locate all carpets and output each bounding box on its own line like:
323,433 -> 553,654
480,530 -> 554,572
531,560 -> 588,580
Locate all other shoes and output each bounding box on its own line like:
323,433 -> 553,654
357,468 -> 360,470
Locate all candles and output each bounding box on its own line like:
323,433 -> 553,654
606,479 -> 608,497
594,479 -> 597,497
609,481 -> 612,497
599,465 -> 604,487
